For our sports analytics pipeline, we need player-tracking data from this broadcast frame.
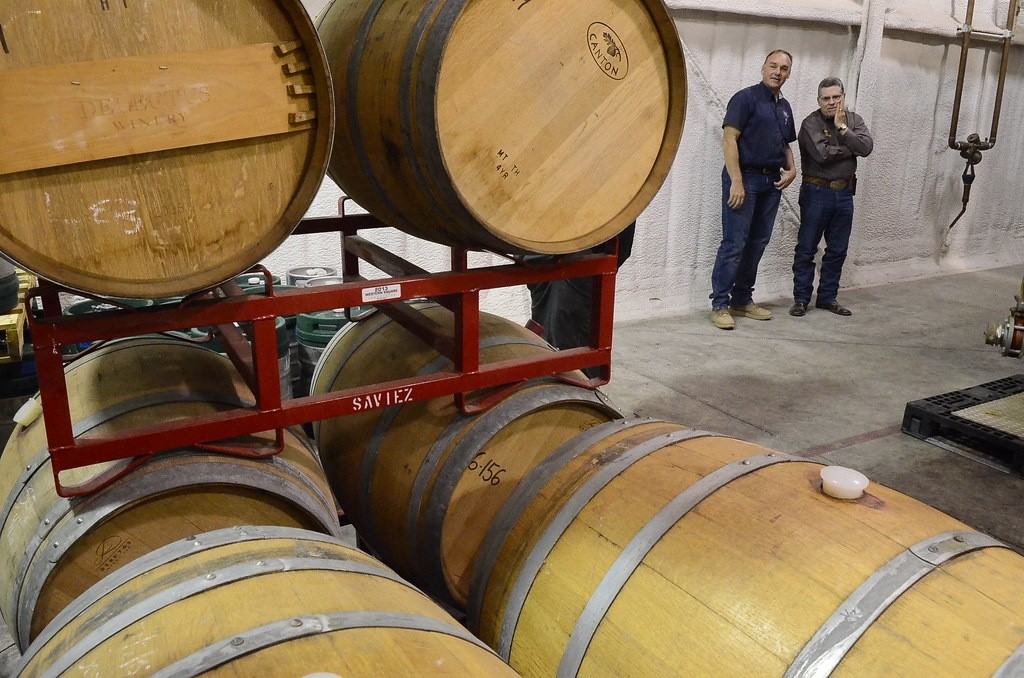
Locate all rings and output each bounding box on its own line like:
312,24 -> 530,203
840,109 -> 843,111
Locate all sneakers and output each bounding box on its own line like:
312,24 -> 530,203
728,302 -> 773,320
710,310 -> 736,329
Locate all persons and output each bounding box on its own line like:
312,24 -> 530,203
789,76 -> 873,316
709,50 -> 797,329
514,218 -> 637,381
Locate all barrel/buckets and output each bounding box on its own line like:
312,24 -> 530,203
0,0 -> 1024,678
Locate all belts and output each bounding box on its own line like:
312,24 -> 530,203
802,175 -> 831,186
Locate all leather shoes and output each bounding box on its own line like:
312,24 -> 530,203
789,302 -> 805,317
816,301 -> 852,316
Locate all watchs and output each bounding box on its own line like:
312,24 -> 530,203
838,123 -> 847,132
